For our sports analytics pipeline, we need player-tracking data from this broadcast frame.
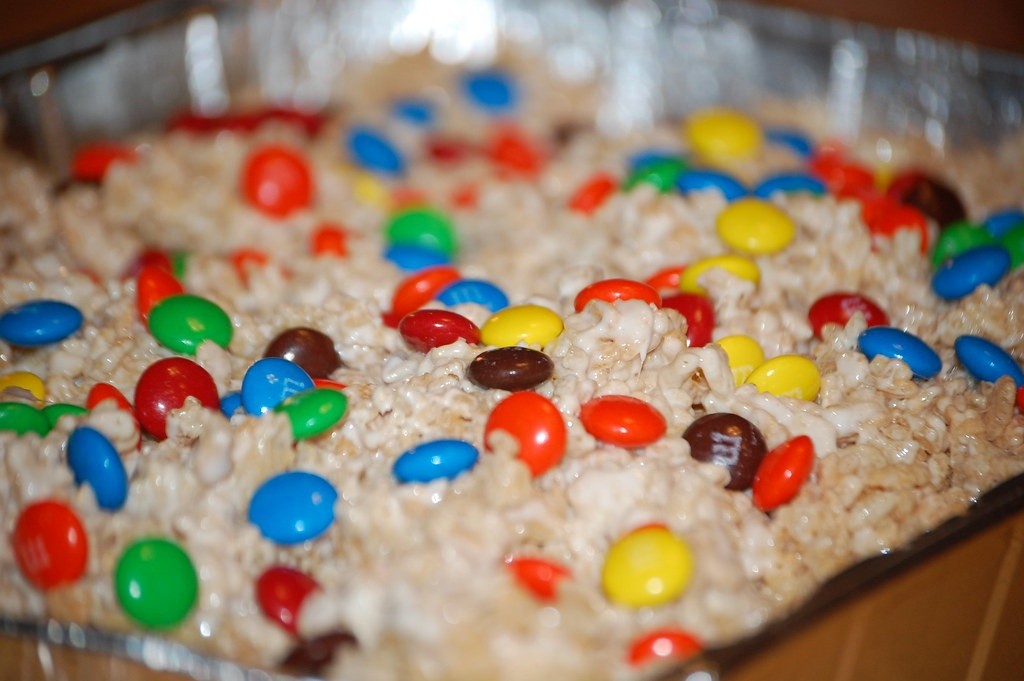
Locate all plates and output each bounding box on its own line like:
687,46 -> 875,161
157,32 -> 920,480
0,0 -> 1024,681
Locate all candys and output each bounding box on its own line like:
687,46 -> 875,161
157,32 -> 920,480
0,72 -> 1024,665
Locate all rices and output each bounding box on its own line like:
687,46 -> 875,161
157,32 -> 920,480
0,85 -> 1024,681
